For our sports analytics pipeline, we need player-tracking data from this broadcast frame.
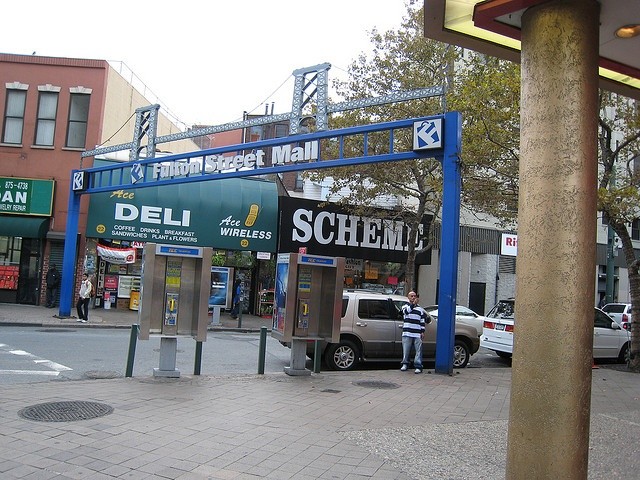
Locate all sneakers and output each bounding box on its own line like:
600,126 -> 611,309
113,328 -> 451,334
82,320 -> 88,323
401,364 -> 408,370
415,368 -> 422,373
77,319 -> 83,322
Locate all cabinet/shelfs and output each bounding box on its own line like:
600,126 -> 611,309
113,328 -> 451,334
256,290 -> 275,317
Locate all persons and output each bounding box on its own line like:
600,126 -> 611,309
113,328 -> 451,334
401,291 -> 426,374
43,263 -> 60,308
229,278 -> 241,321
76,273 -> 92,323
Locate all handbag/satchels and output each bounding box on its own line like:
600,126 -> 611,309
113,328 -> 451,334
87,281 -> 96,297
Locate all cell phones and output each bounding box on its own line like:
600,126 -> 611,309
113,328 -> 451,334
416,293 -> 419,300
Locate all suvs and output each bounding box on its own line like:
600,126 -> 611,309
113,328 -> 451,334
279,288 -> 480,372
601,303 -> 632,333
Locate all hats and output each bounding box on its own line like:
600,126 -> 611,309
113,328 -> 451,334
50,263 -> 55,266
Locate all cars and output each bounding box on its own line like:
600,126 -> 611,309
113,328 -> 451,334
480,297 -> 631,364
423,304 -> 486,336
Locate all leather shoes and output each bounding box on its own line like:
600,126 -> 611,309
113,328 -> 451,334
48,305 -> 55,308
